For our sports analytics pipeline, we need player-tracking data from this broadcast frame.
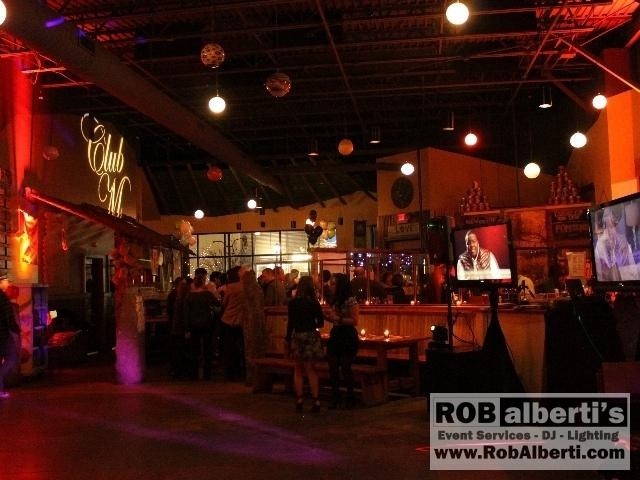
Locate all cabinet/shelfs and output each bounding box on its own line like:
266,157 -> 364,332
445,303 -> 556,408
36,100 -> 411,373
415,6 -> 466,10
11,283 -> 49,377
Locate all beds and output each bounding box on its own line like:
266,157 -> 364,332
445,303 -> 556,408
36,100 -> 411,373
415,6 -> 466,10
268,334 -> 432,397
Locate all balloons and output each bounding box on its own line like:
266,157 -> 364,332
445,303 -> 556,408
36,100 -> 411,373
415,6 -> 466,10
172,218 -> 200,249
304,208 -> 337,248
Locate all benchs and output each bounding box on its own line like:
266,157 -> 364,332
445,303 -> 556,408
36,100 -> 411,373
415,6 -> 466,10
250,358 -> 387,407
356,353 -> 426,364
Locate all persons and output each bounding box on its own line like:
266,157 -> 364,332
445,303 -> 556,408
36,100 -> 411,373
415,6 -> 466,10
0,288 -> 22,400
532,259 -> 571,295
595,206 -> 639,281
455,228 -> 501,275
164,265 -> 455,416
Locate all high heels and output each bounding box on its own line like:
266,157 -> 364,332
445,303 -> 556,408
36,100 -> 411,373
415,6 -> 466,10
310,397 -> 321,414
293,395 -> 305,410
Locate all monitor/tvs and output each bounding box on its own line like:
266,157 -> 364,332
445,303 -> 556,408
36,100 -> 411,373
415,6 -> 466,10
451,220 -> 518,288
586,192 -> 640,291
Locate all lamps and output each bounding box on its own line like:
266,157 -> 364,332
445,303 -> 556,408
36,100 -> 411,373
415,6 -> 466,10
255,181 -> 263,210
442,91 -> 477,146
338,115 -> 354,155
524,30 -> 607,178
208,74 -> 226,113
445,1 -> 470,26
306,115 -> 319,156
368,90 -> 380,144
